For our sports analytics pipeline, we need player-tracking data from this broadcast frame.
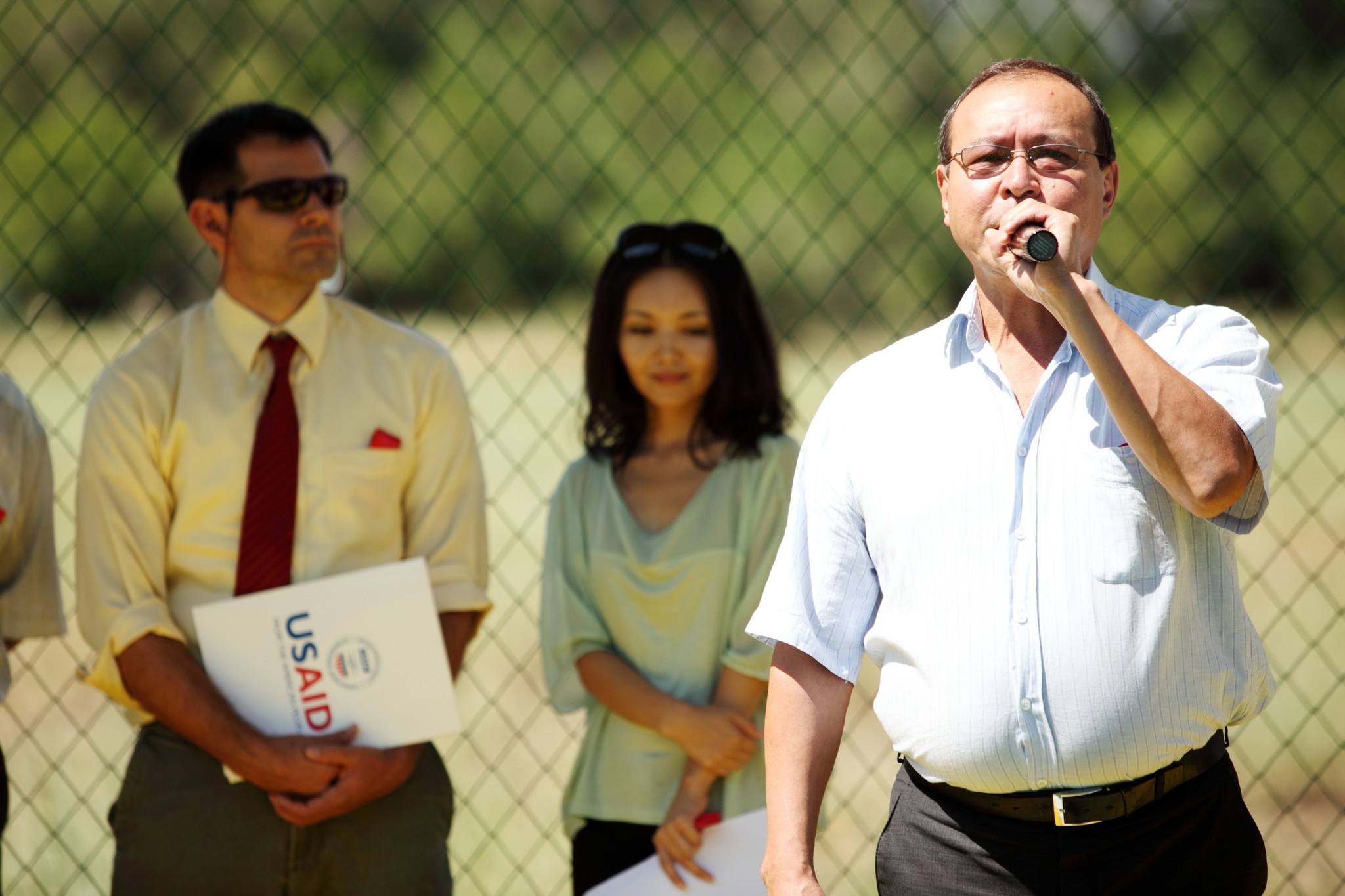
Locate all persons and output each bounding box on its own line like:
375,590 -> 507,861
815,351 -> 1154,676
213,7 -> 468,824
2,369 -> 69,836
740,60 -> 1284,894
76,101 -> 494,895
541,218 -> 798,896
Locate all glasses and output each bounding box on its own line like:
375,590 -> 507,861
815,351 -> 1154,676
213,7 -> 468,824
943,144 -> 1110,171
616,221 -> 726,264
212,173 -> 348,213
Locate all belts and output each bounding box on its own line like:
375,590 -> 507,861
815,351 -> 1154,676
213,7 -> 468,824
934,729 -> 1226,828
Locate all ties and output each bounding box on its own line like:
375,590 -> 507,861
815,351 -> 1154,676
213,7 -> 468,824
234,335 -> 300,597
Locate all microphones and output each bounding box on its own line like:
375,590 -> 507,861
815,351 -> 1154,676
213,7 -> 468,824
1008,221 -> 1060,264
